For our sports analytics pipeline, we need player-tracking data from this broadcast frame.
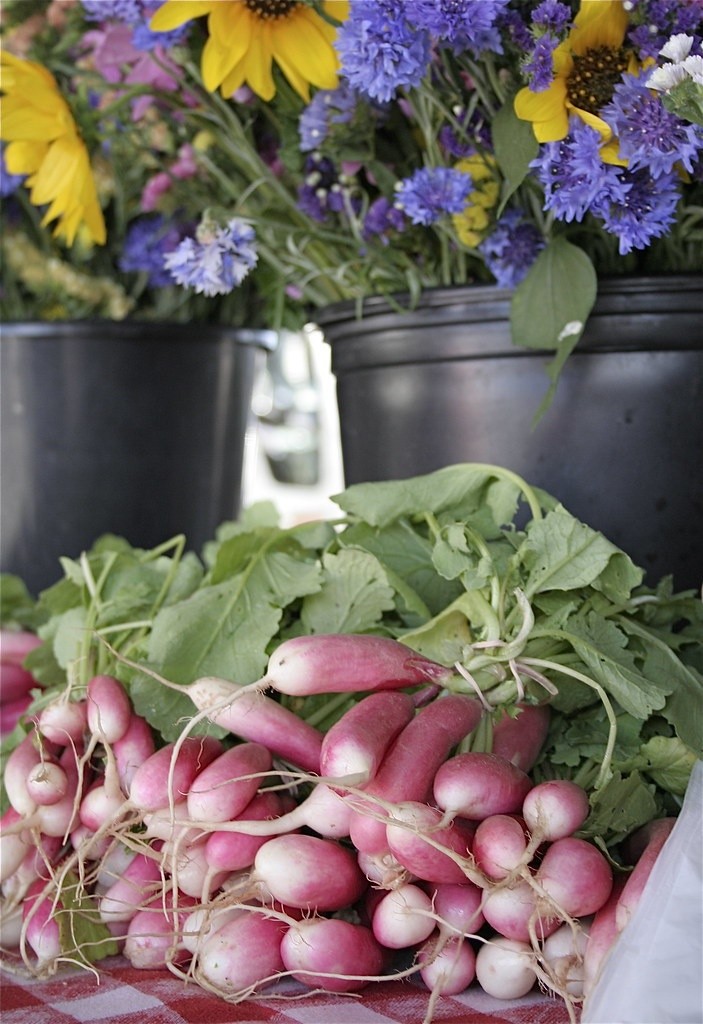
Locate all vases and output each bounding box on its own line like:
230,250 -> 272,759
1,324 -> 278,606
311,278 -> 703,601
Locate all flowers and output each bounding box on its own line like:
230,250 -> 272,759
0,0 -> 703,436
